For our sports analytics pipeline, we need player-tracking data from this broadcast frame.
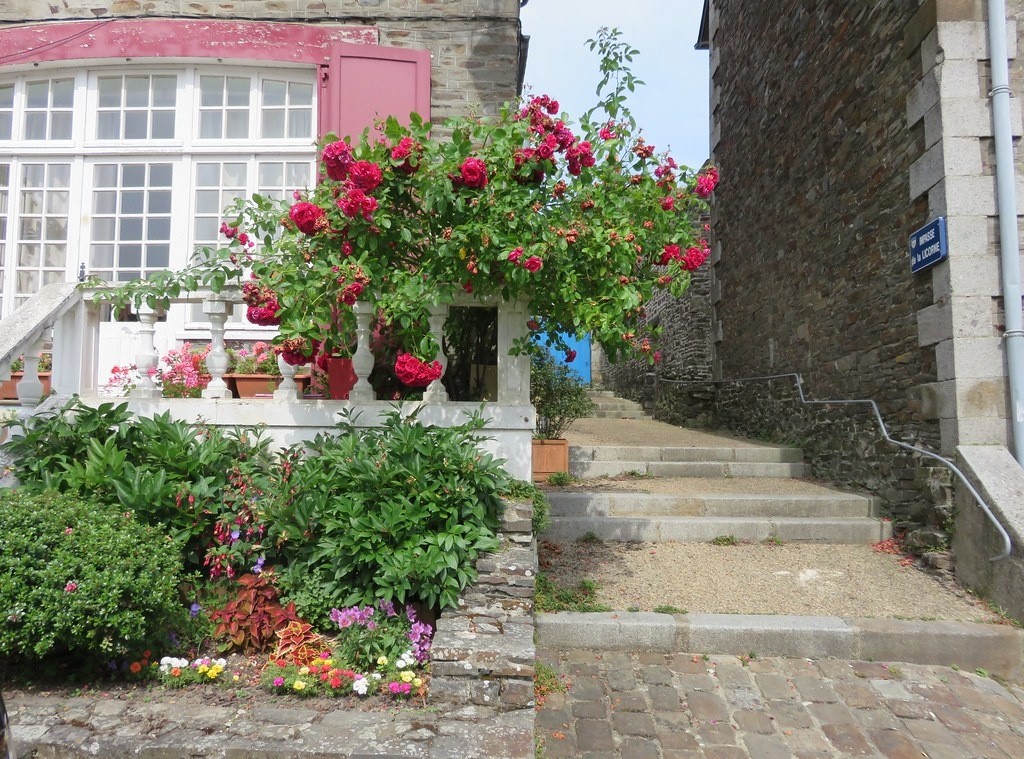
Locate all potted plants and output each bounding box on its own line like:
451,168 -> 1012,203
530,345 -> 599,481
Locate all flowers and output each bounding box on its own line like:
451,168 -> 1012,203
104,341 -> 307,398
11,353 -> 52,373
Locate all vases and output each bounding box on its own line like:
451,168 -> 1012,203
0,371 -> 52,400
204,374 -> 232,390
232,373 -> 310,398
326,358 -> 358,400
